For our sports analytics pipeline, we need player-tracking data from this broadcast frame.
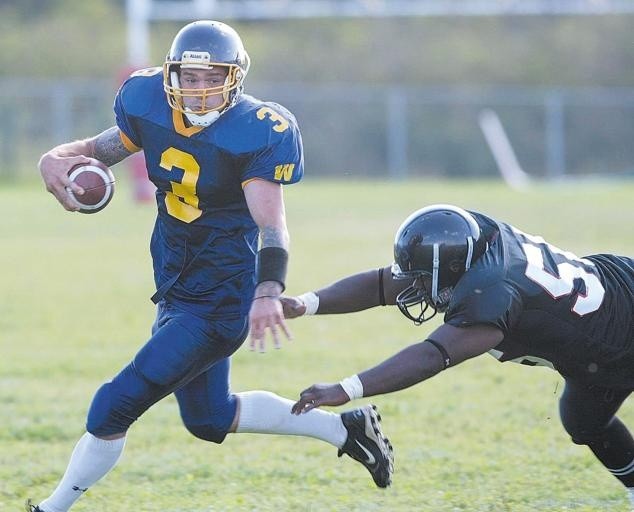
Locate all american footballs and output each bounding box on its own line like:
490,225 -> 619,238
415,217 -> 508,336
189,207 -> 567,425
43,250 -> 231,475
67,156 -> 115,214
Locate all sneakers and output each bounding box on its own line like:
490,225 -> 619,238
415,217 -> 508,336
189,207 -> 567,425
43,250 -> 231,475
337,404 -> 394,489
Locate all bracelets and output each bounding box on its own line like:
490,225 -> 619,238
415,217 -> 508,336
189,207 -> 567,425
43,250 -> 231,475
340,374 -> 365,399
253,248 -> 288,292
296,291 -> 318,317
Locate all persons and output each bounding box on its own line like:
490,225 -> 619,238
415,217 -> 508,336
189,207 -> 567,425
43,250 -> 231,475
271,204 -> 633,499
21,19 -> 394,512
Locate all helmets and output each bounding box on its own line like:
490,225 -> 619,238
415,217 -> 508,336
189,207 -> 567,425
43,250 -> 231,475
391,205 -> 488,323
163,19 -> 250,127
